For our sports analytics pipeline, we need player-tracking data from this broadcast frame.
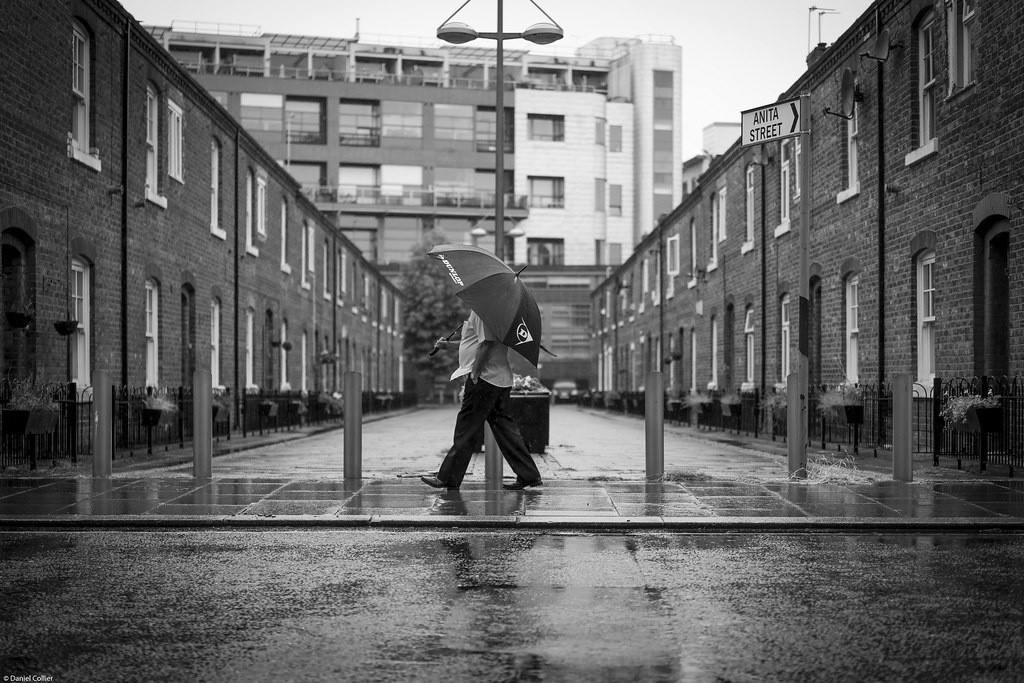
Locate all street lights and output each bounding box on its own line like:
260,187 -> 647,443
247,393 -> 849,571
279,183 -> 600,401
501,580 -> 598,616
435,0 -> 563,480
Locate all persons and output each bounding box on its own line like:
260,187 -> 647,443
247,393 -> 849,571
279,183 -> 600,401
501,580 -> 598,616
421,310 -> 545,491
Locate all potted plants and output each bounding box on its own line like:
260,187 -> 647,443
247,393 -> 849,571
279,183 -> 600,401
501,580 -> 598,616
938,389 -> 1005,431
817,356 -> 864,425
720,391 -> 741,416
6,299 -> 34,329
287,401 -> 301,413
213,394 -> 232,420
1,380 -> 59,433
138,395 -> 176,425
258,399 -> 279,417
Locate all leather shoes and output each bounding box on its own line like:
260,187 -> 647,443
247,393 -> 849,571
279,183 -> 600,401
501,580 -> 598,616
421,475 -> 460,490
504,476 -> 543,490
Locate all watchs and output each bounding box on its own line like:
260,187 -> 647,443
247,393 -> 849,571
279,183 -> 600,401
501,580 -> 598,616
470,374 -> 478,380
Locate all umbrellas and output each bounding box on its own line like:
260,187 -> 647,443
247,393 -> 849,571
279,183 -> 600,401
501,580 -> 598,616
427,244 -> 558,370
